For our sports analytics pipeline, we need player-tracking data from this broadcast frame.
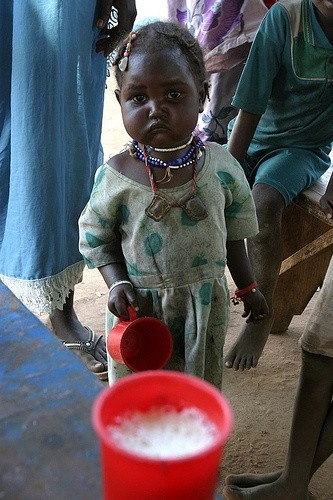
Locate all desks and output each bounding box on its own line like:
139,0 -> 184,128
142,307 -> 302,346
0,278 -> 105,500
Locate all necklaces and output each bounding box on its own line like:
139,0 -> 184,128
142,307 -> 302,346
129,133 -> 210,221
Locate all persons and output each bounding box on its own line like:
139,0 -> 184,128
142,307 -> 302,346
221,0 -> 333,372
224,249 -> 333,500
0,0 -> 138,381
167,0 -> 278,145
78,22 -> 268,399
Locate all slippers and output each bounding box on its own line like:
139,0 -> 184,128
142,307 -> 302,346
59,325 -> 108,379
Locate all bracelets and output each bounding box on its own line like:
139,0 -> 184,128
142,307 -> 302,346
109,281 -> 134,294
230,282 -> 257,306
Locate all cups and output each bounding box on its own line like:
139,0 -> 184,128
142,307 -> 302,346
106,304 -> 173,374
91,370 -> 234,500
